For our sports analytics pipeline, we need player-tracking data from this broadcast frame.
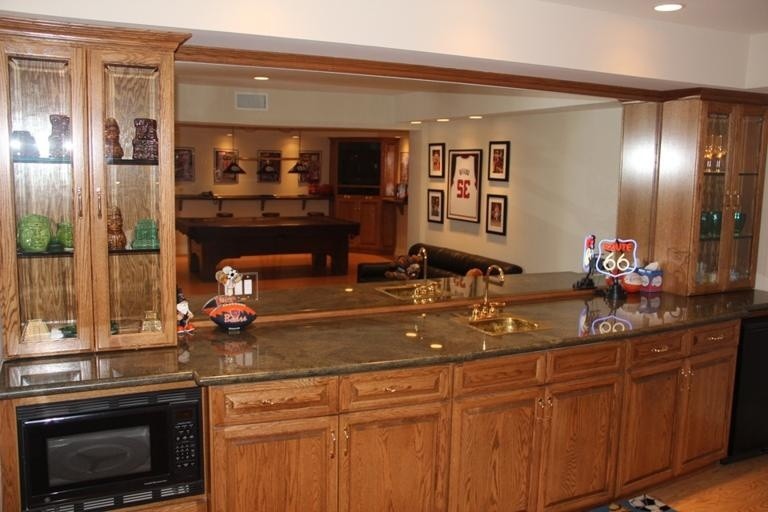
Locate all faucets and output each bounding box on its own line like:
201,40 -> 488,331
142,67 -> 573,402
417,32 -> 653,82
470,264 -> 507,320
414,248 -> 446,303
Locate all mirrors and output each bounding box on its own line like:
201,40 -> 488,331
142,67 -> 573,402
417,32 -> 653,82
173,47 -> 662,332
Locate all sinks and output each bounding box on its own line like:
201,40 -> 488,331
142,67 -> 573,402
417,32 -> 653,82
450,315 -> 553,337
374,286 -> 417,298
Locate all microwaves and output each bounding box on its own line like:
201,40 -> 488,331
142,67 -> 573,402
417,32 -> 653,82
16,386 -> 204,512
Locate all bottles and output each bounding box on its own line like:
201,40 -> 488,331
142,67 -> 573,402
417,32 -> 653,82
142,310 -> 161,332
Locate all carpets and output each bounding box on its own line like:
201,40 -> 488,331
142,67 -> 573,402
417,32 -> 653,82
448,339 -> 624,512
581,495 -> 675,512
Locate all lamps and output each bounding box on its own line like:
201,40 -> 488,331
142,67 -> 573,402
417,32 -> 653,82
288,134 -> 309,173
224,128 -> 248,175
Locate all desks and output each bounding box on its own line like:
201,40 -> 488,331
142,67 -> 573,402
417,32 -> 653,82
175,215 -> 361,283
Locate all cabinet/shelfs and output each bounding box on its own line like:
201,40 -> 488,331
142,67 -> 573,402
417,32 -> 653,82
1,19 -> 193,361
653,96 -> 768,298
326,138 -> 405,253
613,315 -> 742,501
0,358 -> 453,512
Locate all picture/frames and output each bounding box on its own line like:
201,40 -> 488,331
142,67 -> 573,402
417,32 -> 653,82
428,143 -> 444,177
447,149 -> 482,223
486,194 -> 506,235
488,141 -> 509,181
427,189 -> 444,223
174,147 -> 322,182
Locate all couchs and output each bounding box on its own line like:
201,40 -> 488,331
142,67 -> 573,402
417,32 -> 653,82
358,243 -> 523,283
